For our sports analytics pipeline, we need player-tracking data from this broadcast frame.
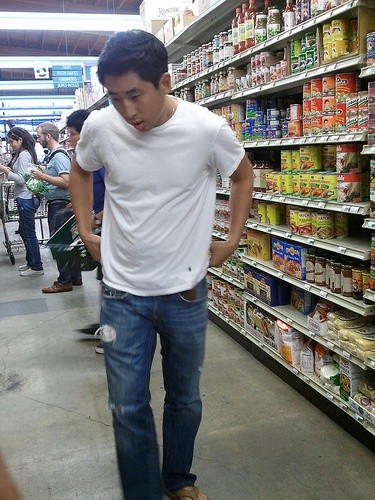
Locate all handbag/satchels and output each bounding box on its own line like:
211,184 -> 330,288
26,164 -> 45,193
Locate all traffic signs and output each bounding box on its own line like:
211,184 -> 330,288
51,64 -> 85,89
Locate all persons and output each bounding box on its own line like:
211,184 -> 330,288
67,28 -> 254,500
65,109 -> 107,353
30,121 -> 82,293
0,127 -> 42,276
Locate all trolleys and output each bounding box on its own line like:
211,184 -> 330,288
0,176 -> 52,266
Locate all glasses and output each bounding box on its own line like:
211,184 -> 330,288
8,131 -> 20,138
35,133 -> 46,138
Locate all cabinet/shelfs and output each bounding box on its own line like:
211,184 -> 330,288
58,0 -> 375,455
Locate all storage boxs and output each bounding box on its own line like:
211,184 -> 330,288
246,229 -> 270,260
285,242 -> 308,280
290,285 -> 310,315
242,265 -> 255,295
278,280 -> 285,306
155,27 -> 164,44
190,0 -> 210,18
174,4 -> 193,36
340,358 -> 365,403
138,0 -> 191,35
164,18 -> 175,46
255,271 -> 278,307
272,238 -> 286,273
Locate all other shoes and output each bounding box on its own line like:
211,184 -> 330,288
78,324 -> 100,335
95,344 -> 104,354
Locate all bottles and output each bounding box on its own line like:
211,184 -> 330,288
174,67 -> 234,101
285,0 -> 295,31
231,0 -> 282,55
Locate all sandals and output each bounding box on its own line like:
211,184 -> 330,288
164,485 -> 208,500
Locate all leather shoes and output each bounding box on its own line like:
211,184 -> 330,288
42,281 -> 73,293
73,280 -> 83,285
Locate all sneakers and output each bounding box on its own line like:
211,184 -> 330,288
18,264 -> 29,270
20,268 -> 44,276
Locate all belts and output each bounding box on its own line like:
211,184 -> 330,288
50,200 -> 68,204
